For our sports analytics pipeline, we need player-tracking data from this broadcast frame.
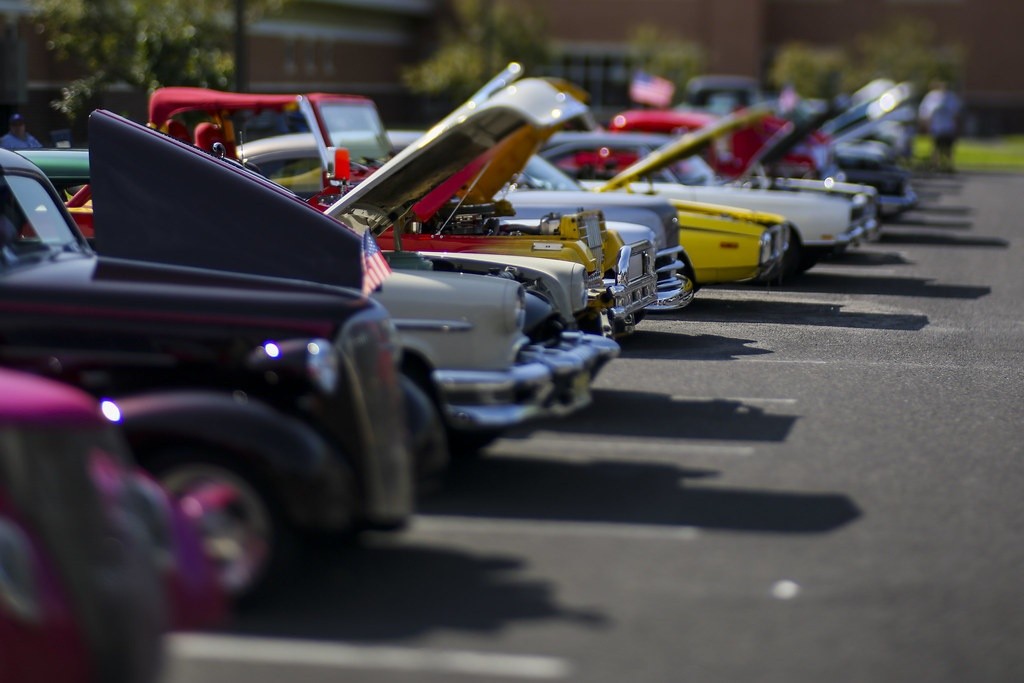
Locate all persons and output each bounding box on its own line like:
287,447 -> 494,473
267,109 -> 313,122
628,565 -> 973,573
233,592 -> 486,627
0,114 -> 43,157
920,80 -> 966,171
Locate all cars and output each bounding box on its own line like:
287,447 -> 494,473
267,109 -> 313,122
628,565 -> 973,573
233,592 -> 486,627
1,61 -> 938,683
